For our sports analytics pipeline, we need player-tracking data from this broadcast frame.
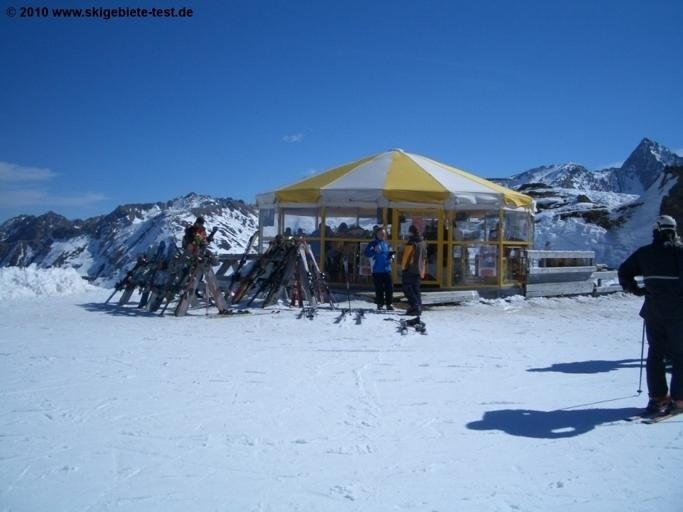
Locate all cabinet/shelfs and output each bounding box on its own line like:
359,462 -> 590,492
359,243 -> 375,276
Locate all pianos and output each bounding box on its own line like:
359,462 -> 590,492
102,224 -> 336,318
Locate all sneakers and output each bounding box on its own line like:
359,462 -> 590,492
377,304 -> 395,310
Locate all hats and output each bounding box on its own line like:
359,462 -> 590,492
195,216 -> 206,225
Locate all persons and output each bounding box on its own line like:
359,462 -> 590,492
183,216 -> 207,252
617,215 -> 682,416
283,219 -> 465,316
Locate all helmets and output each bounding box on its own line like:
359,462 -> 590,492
654,214 -> 677,232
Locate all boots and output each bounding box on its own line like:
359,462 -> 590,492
645,396 -> 683,415
407,304 -> 422,315
